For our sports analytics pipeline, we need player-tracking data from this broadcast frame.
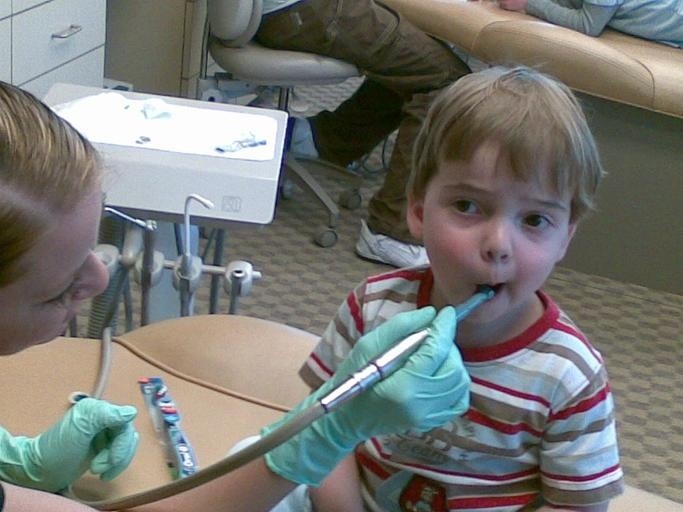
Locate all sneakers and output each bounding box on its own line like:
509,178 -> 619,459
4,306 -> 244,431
355,218 -> 431,269
287,116 -> 320,161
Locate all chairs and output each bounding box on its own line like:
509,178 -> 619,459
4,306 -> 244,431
203,2 -> 364,247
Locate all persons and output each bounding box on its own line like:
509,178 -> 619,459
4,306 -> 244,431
295,56 -> 628,511
497,1 -> 682,53
0,80 -> 471,512
255,1 -> 471,271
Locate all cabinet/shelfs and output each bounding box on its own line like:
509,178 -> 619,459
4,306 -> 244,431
0,1 -> 108,108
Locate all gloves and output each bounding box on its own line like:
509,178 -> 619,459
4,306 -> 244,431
260,304 -> 472,487
0,398 -> 139,492
